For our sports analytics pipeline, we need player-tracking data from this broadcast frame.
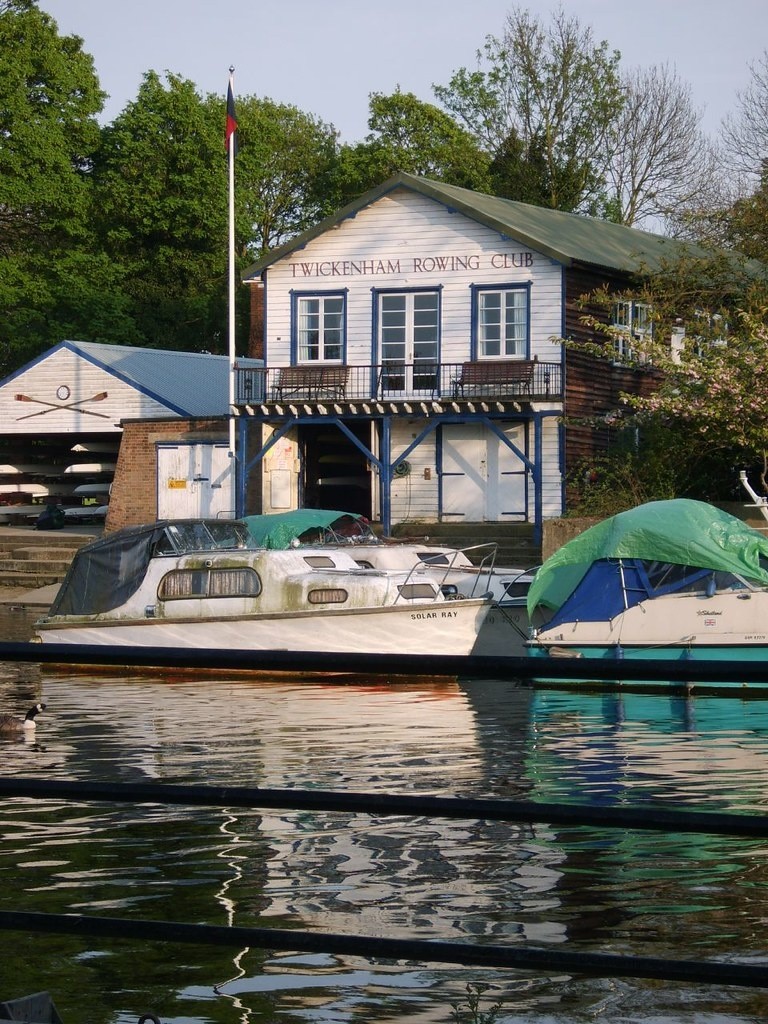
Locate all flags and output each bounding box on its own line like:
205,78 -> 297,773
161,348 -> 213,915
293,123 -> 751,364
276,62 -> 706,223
225,65 -> 240,156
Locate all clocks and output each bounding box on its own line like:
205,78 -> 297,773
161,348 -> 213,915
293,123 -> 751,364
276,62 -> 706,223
57,386 -> 70,399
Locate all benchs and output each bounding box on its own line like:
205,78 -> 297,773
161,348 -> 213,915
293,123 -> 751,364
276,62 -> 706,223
271,365 -> 351,399
453,359 -> 538,393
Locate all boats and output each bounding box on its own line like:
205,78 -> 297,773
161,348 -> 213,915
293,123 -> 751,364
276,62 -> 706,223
27,508 -> 536,678
493,468 -> 767,693
